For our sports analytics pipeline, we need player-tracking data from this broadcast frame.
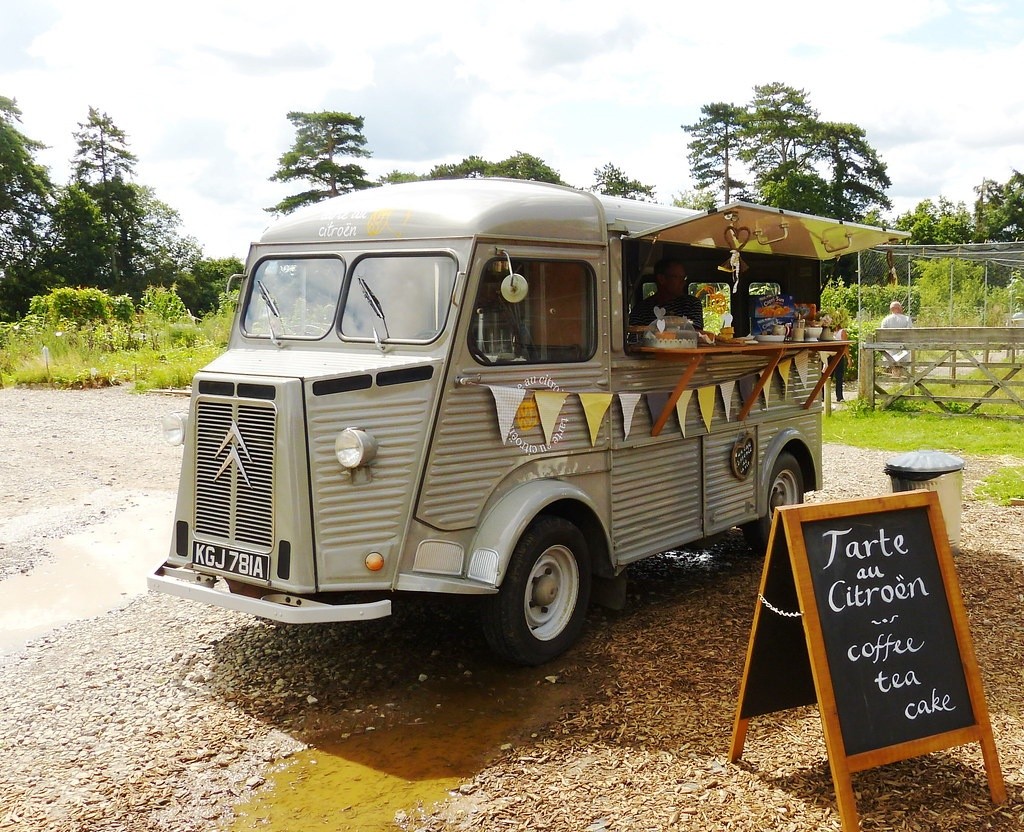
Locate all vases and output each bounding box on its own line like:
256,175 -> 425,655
817,326 -> 836,341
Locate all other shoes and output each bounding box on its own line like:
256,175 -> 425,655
839,399 -> 845,402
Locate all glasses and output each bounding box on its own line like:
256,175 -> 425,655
669,274 -> 688,284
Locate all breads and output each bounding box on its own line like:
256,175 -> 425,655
696,330 -> 714,341
655,332 -> 676,340
718,326 -> 744,344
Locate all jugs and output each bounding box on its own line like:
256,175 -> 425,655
772,325 -> 789,340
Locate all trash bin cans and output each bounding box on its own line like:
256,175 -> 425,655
881,450 -> 965,556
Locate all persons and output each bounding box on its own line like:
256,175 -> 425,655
821,314 -> 851,402
881,301 -> 914,377
628,258 -> 704,343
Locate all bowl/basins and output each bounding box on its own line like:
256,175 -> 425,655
805,327 -> 822,342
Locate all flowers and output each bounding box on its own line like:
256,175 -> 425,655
817,307 -> 840,327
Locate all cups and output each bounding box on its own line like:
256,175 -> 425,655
792,319 -> 805,341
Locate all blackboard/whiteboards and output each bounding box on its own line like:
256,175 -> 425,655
781,490 -> 993,770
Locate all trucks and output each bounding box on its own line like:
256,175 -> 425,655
146,175 -> 917,671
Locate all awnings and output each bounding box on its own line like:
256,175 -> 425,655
628,201 -> 911,260
